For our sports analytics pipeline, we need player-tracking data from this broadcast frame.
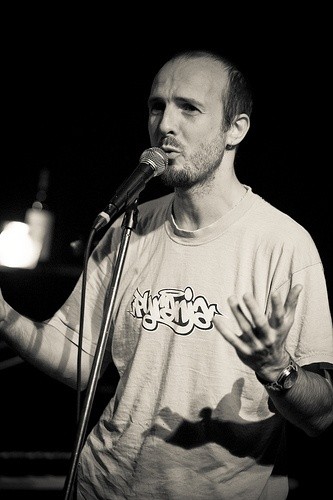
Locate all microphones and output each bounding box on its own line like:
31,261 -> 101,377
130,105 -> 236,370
92,148 -> 168,232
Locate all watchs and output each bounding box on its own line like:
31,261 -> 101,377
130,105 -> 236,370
257,352 -> 298,393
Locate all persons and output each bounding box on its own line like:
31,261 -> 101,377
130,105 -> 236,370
0,49 -> 333,500
